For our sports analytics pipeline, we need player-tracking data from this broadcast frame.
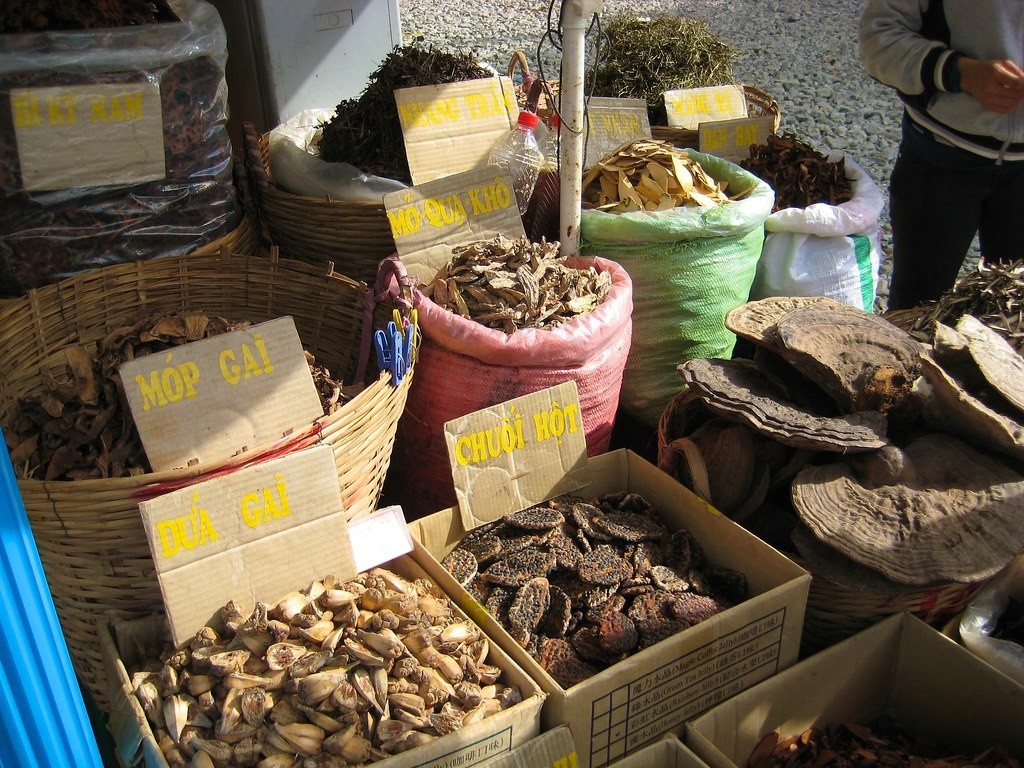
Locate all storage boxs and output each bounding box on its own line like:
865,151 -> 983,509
95,556 -> 550,768
685,606 -> 1024,768
485,723 -> 711,768
406,447 -> 814,768
959,555 -> 1024,682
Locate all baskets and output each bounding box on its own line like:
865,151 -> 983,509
242,117 -> 545,288
656,387 -> 987,658
508,49 -> 781,150
0,244 -> 418,713
0,144 -> 263,309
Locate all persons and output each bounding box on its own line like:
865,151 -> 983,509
858,0 -> 1024,312
